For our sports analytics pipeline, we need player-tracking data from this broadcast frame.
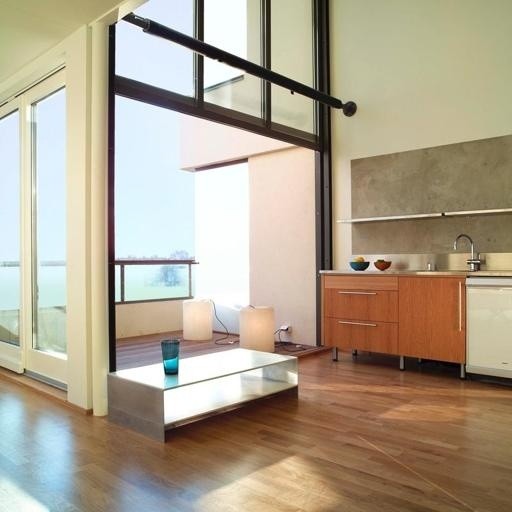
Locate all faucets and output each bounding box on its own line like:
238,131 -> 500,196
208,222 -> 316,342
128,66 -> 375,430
454,233 -> 474,261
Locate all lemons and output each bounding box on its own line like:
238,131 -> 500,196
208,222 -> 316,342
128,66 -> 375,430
353,256 -> 365,262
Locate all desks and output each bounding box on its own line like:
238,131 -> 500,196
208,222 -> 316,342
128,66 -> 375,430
107,344 -> 305,444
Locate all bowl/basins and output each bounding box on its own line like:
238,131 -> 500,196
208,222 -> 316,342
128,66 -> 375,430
374,261 -> 392,271
349,262 -> 370,271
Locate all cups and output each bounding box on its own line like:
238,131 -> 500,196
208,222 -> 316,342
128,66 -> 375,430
161,340 -> 179,375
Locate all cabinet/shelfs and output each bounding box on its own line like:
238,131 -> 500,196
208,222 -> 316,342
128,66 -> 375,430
319,276 -> 398,356
467,285 -> 511,376
400,279 -> 467,365
